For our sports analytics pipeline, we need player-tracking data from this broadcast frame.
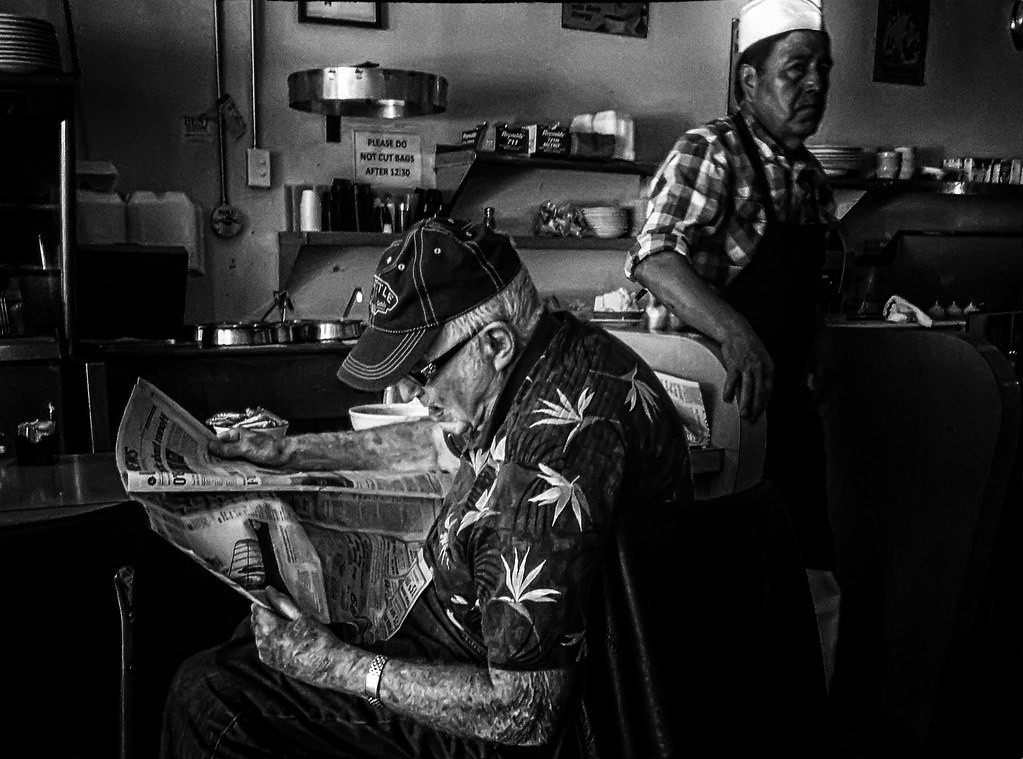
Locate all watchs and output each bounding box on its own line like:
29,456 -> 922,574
362,655 -> 391,711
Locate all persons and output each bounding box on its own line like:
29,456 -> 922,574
623,0 -> 845,689
155,214 -> 699,759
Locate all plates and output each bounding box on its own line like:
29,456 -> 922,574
805,145 -> 864,176
582,200 -> 648,238
0,13 -> 64,71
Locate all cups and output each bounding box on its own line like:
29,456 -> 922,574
894,146 -> 917,179
875,150 -> 901,179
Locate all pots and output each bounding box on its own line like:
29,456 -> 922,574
195,319 -> 364,346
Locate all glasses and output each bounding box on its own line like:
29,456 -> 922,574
397,325 -> 501,390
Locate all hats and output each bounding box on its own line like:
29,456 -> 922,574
737,0 -> 829,54
338,216 -> 522,392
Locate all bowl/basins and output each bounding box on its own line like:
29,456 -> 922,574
349,404 -> 430,431
589,308 -> 645,319
589,319 -> 644,332
213,420 -> 288,440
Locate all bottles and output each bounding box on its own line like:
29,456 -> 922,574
295,178 -> 446,233
483,207 -> 495,230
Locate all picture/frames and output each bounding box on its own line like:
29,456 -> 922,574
297,0 -> 383,29
